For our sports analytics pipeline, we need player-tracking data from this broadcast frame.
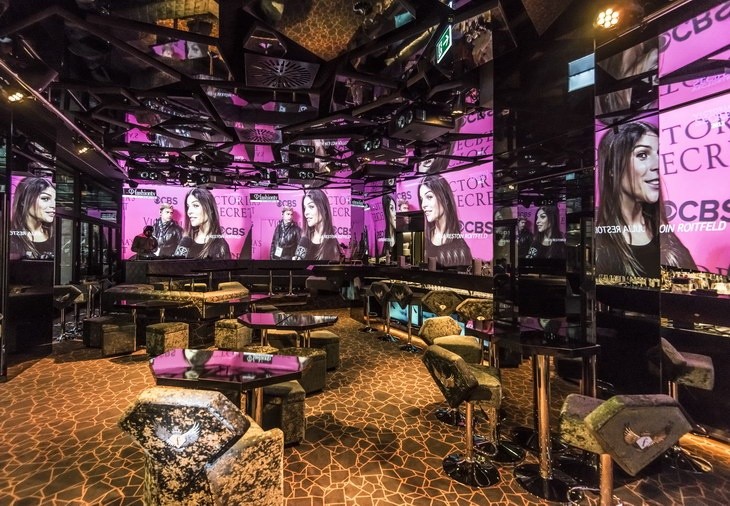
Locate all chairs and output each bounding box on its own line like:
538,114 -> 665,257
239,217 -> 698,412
53,274 -> 714,506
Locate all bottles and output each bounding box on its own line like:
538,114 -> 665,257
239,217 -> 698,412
660,268 -> 723,290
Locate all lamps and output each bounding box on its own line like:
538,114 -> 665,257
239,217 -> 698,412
3,85 -> 24,102
591,6 -> 627,32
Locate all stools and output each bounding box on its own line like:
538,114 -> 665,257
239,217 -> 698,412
260,328 -> 299,349
258,380 -> 305,447
81,314 -> 116,349
146,321 -> 191,358
102,321 -> 137,355
269,348 -> 327,393
214,319 -> 253,350
255,305 -> 277,312
184,283 -> 208,292
301,331 -> 340,370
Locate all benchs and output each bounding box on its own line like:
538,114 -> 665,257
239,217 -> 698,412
102,281 -> 248,323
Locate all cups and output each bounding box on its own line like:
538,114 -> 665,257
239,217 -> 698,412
13,287 -> 21,294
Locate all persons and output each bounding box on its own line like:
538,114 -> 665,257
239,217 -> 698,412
131,226 -> 158,259
415,119 -> 472,265
494,205 -> 565,265
381,195 -> 410,257
152,41 -> 231,259
593,36 -> 699,276
493,259 -> 510,275
269,94 -> 341,260
8,178 -> 56,259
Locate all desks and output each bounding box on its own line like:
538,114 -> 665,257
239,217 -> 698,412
491,331 -> 602,502
206,293 -> 271,319
184,273 -> 207,291
237,313 -> 337,349
112,299 -> 194,353
147,346 -> 312,428
